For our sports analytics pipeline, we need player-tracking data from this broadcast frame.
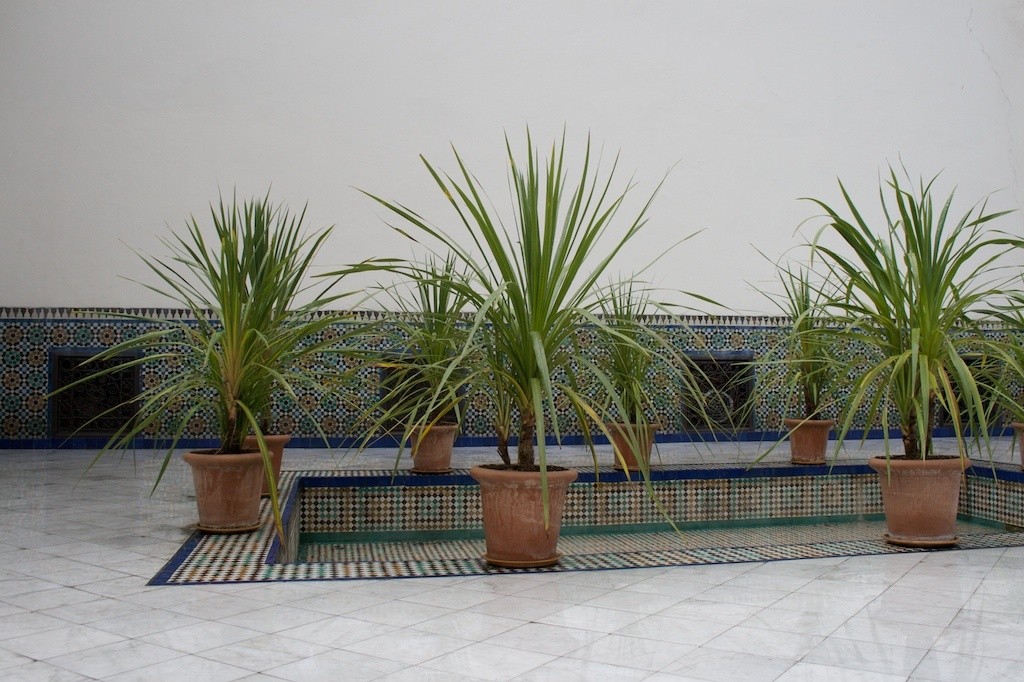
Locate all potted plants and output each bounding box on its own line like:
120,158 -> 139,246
45,123 -> 1024,568
